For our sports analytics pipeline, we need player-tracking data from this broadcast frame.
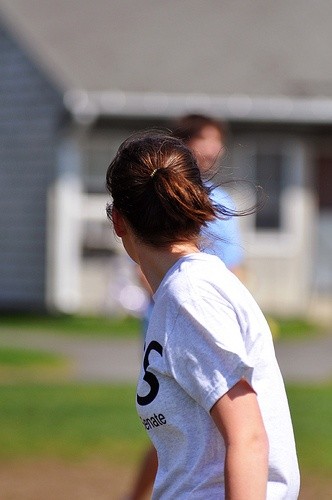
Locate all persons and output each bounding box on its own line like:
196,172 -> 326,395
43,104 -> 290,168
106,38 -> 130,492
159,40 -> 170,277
126,114 -> 252,499
104,132 -> 303,500
